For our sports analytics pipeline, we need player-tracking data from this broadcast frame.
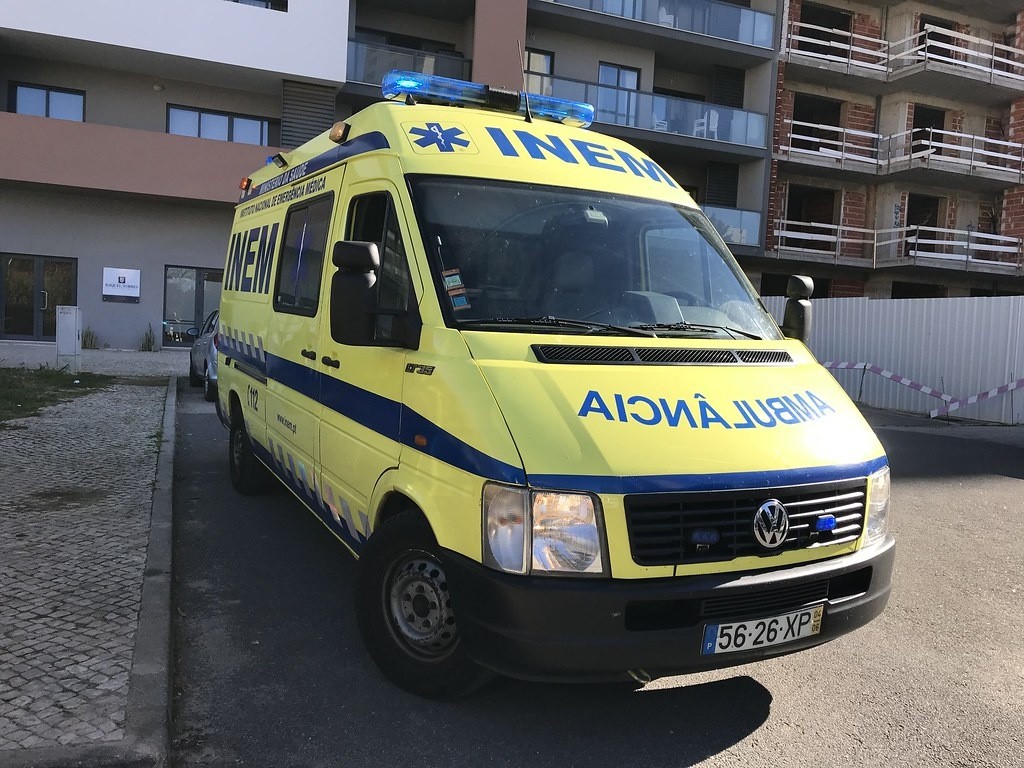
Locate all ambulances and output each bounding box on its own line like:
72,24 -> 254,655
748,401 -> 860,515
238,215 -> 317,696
210,68 -> 899,695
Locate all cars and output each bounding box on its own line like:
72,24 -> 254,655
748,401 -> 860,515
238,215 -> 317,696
185,309 -> 220,403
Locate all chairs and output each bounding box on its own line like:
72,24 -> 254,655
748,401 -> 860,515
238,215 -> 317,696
371,211 -> 411,339
534,216 -> 625,317
659,7 -> 679,29
693,109 -> 719,139
651,112 -> 667,131
